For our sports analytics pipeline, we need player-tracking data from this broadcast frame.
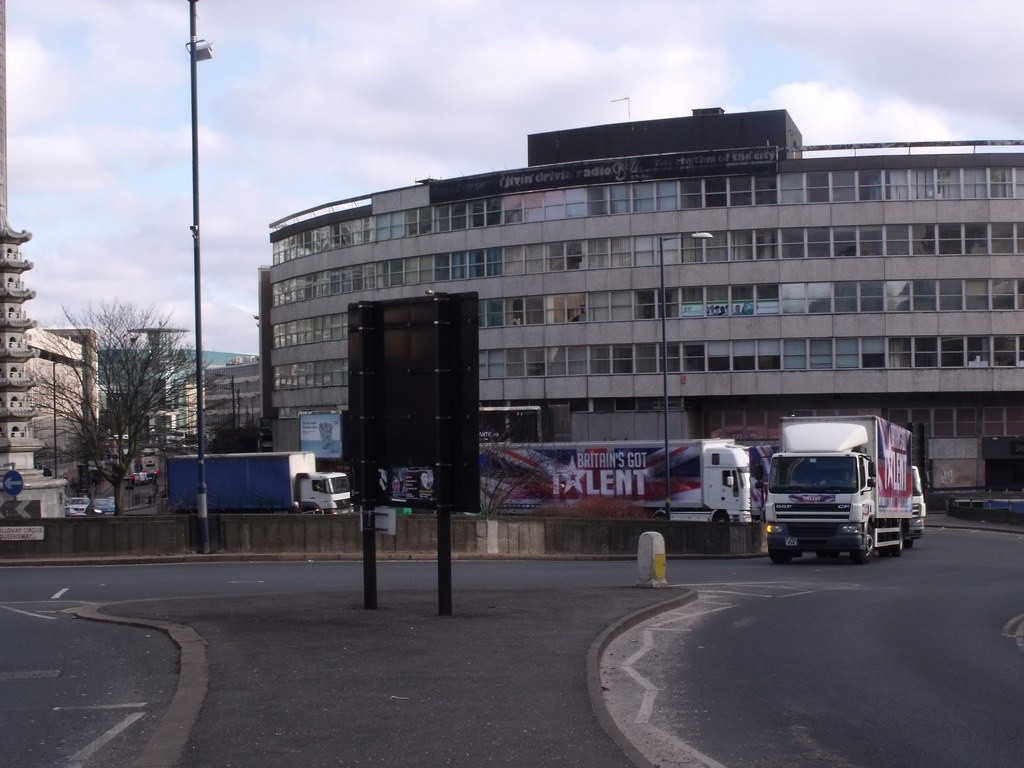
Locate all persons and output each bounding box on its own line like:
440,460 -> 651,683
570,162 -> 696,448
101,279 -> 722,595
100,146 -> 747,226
707,305 -> 743,315
313,481 -> 321,491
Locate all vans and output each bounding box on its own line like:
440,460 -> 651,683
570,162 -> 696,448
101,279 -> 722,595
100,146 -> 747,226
132,472 -> 152,484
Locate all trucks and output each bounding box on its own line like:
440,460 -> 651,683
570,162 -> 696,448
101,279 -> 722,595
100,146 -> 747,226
755,414 -> 913,565
908,465 -> 933,539
167,452 -> 354,515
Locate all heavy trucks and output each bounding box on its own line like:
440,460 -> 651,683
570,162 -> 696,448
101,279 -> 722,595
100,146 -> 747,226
749,446 -> 782,519
477,439 -> 756,524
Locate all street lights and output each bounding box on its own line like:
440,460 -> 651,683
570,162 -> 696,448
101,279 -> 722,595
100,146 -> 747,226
52,359 -> 63,478
660,233 -> 715,518
187,38 -> 215,551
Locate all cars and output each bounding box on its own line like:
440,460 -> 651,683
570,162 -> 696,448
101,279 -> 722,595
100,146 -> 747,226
94,499 -> 116,516
66,498 -> 90,516
